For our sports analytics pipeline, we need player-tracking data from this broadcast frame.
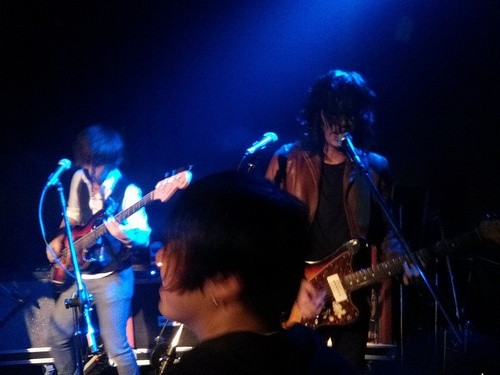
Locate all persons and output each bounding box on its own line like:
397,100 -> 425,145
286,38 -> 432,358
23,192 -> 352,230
154,171 -> 355,375
265,70 -> 426,375
46,125 -> 152,375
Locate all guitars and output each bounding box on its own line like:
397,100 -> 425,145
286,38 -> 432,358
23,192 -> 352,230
50,165 -> 192,293
280,213 -> 500,330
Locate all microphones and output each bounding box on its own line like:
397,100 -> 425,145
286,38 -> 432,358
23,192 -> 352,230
46,158 -> 71,188
338,132 -> 367,174
244,131 -> 278,158
